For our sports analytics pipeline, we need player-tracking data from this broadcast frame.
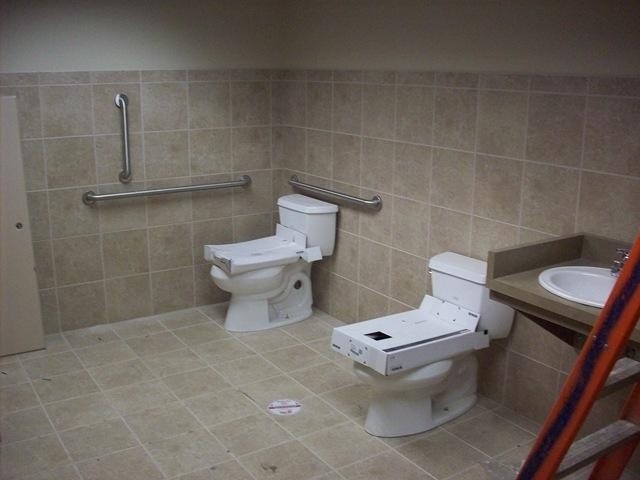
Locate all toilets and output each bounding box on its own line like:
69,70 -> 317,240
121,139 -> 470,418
210,193 -> 340,334
353,251 -> 517,437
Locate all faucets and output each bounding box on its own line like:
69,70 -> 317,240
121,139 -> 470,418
611,249 -> 627,275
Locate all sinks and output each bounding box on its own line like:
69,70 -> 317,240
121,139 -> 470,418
537,265 -> 619,307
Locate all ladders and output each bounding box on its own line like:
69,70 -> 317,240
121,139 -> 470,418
516,233 -> 640,480
